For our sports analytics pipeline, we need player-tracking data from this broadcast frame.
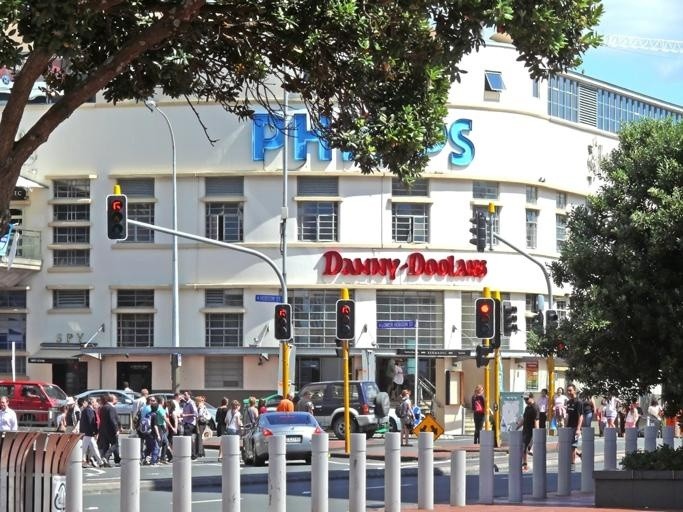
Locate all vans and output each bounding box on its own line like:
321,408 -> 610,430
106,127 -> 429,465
0,380 -> 68,430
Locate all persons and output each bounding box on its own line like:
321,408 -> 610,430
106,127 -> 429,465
0,394 -> 18,452
395,389 -> 416,447
512,383 -> 662,471
471,384 -> 485,444
385,358 -> 404,402
57,381 -> 315,469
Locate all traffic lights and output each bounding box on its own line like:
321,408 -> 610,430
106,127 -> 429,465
544,310 -> 558,337
474,345 -> 489,367
500,301 -> 517,338
468,210 -> 485,250
531,312 -> 543,340
335,298 -> 354,341
105,193 -> 127,242
273,303 -> 291,342
474,298 -> 494,338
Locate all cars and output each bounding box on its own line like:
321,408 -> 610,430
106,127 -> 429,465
634,413 -> 661,439
352,393 -> 425,432
66,388 -> 134,430
242,393 -> 290,421
127,391 -> 220,429
239,410 -> 326,465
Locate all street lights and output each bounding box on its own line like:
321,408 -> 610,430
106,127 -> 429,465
139,100 -> 184,350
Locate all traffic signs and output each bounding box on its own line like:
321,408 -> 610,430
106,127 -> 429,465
253,294 -> 280,303
376,319 -> 416,329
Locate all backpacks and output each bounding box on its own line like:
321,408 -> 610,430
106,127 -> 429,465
395,403 -> 406,417
133,410 -> 152,436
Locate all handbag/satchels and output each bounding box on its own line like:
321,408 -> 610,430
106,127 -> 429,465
474,398 -> 484,413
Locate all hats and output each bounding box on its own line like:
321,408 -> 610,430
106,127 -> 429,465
523,391 -> 533,400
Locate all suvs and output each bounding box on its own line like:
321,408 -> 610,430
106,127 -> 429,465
265,379 -> 389,441
